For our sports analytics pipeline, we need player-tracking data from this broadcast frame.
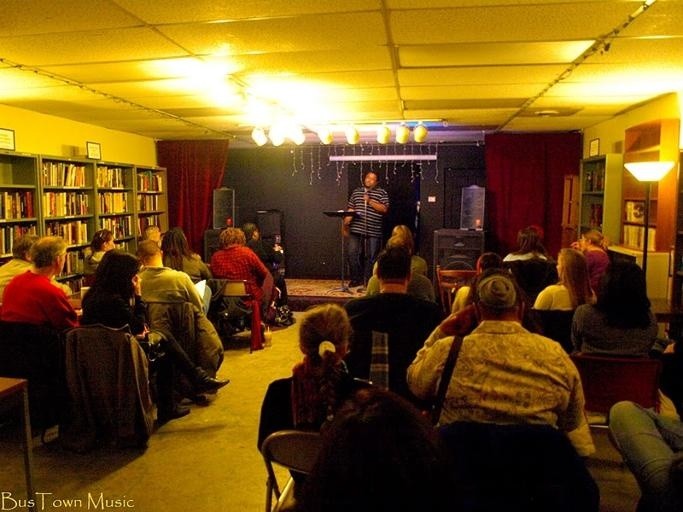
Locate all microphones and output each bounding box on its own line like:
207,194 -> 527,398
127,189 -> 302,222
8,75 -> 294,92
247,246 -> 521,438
361,183 -> 367,192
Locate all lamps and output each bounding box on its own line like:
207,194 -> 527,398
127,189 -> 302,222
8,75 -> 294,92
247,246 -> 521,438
625,160 -> 672,267
248,126 -> 430,145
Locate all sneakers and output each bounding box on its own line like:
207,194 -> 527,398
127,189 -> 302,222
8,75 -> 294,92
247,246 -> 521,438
349,280 -> 364,287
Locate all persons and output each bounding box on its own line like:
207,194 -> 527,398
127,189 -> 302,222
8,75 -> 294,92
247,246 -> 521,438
0,222 -> 683,512
341,170 -> 390,288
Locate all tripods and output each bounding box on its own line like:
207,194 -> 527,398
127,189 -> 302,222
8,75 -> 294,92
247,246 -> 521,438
331,216 -> 353,295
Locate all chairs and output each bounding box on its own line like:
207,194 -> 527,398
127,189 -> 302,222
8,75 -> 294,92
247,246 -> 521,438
204,187 -> 236,258
257,268 -> 683,512
0,262 -> 269,506
452,185 -> 486,231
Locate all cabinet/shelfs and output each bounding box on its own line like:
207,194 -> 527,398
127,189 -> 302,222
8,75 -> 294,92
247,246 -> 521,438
618,118 -> 673,252
433,227 -> 480,270
38,155 -> 92,283
136,169 -> 167,235
578,157 -> 607,238
0,148 -> 41,262
95,163 -> 132,247
560,173 -> 578,228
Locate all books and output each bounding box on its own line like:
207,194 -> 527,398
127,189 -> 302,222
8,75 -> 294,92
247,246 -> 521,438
583,170 -> 659,252
0,151 -> 164,299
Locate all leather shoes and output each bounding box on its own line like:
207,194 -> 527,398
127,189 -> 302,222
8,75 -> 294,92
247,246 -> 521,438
157,407 -> 190,423
193,378 -> 229,395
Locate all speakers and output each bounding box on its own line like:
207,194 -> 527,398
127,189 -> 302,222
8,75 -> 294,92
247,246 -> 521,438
204,229 -> 224,263
211,186 -> 235,230
432,228 -> 485,299
460,186 -> 486,231
